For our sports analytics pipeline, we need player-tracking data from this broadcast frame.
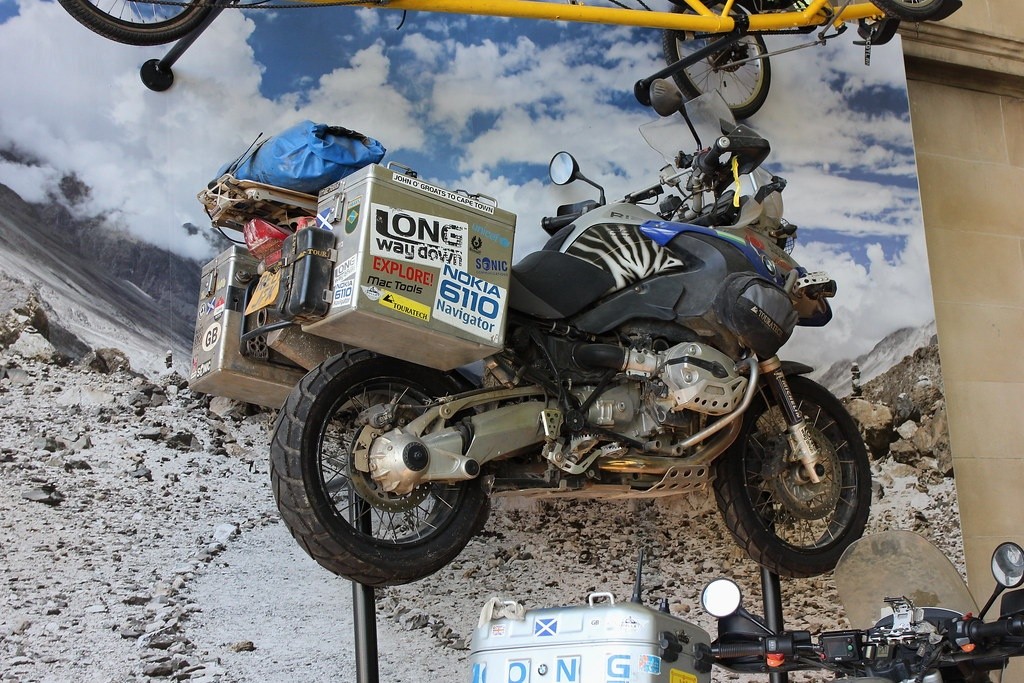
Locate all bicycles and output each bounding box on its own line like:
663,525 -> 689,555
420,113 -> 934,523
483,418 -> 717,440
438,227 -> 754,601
47,1 -> 967,123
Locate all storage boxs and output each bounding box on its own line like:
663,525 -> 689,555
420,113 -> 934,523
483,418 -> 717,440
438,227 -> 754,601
189,245 -> 309,410
466,550 -> 717,683
301,161 -> 517,371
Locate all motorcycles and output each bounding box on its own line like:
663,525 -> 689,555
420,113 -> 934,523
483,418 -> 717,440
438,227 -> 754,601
466,539 -> 1023,683
187,76 -> 874,585
